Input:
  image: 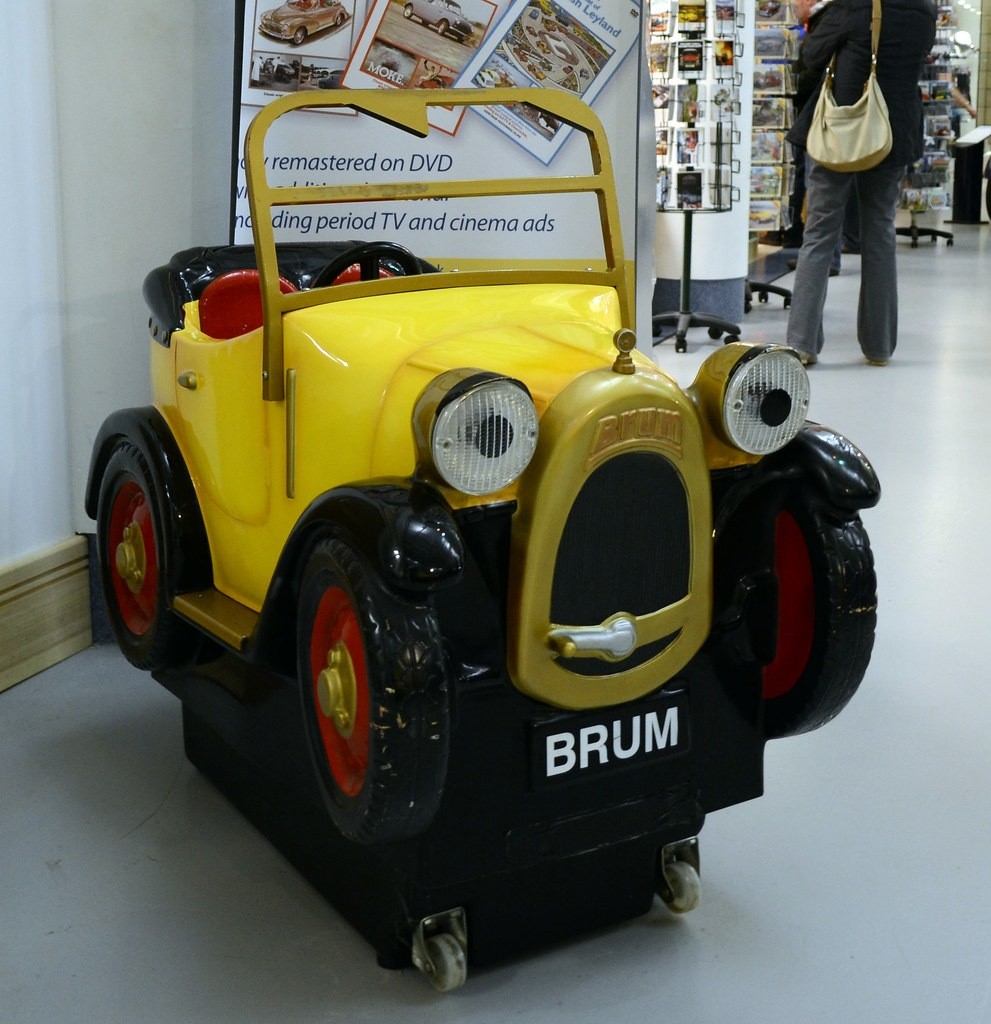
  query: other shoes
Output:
[841,244,858,253]
[870,360,887,366]
[786,258,839,276]
[796,349,818,365]
[759,234,802,247]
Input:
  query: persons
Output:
[759,0,937,365]
[946,82,978,118]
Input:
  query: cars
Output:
[83,86,880,852]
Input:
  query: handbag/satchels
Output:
[806,69,892,173]
